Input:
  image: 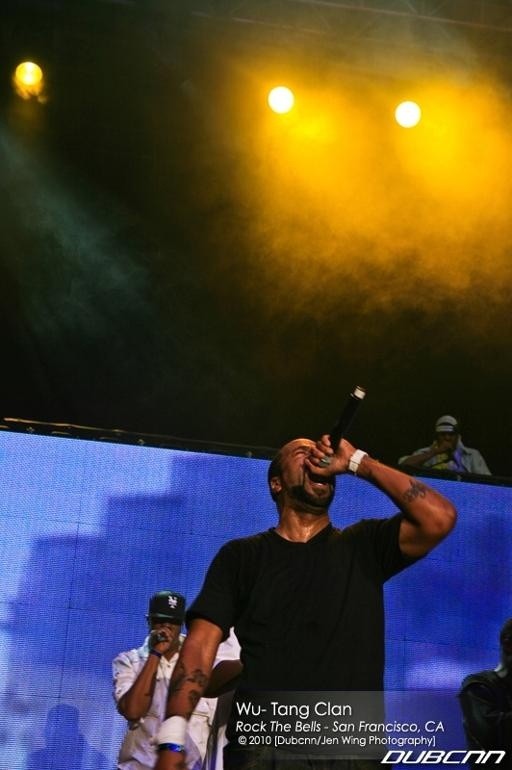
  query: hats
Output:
[143,590,187,625]
[434,413,459,434]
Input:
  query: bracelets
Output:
[149,648,162,659]
[154,715,190,745]
[154,743,189,757]
[347,448,369,477]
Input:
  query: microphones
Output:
[153,632,166,643]
[319,386,366,466]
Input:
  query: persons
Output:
[111,587,242,770]
[153,431,458,770]
[396,413,495,483]
[458,621,511,769]
